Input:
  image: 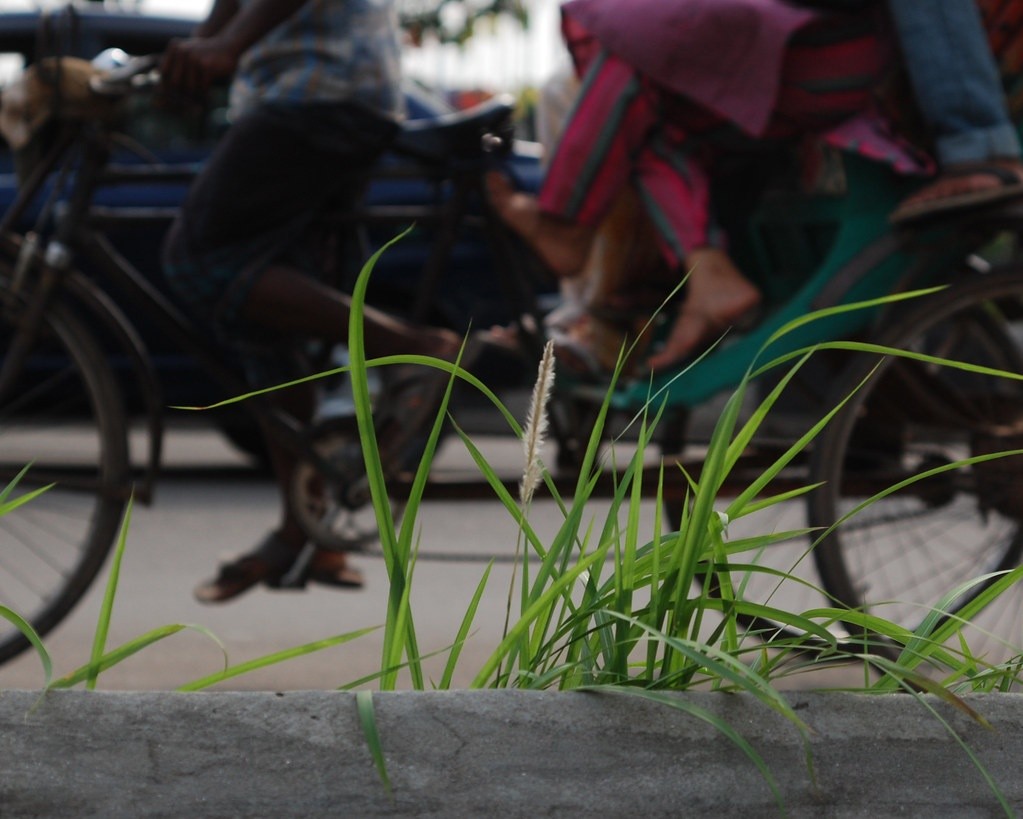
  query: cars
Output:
[1,8,554,415]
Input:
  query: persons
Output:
[484,0,1023,371]
[151,0,470,606]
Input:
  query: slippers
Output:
[340,334,480,515]
[194,521,369,606]
[884,161,1023,225]
[631,303,766,381]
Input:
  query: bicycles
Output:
[0,76,1023,671]
